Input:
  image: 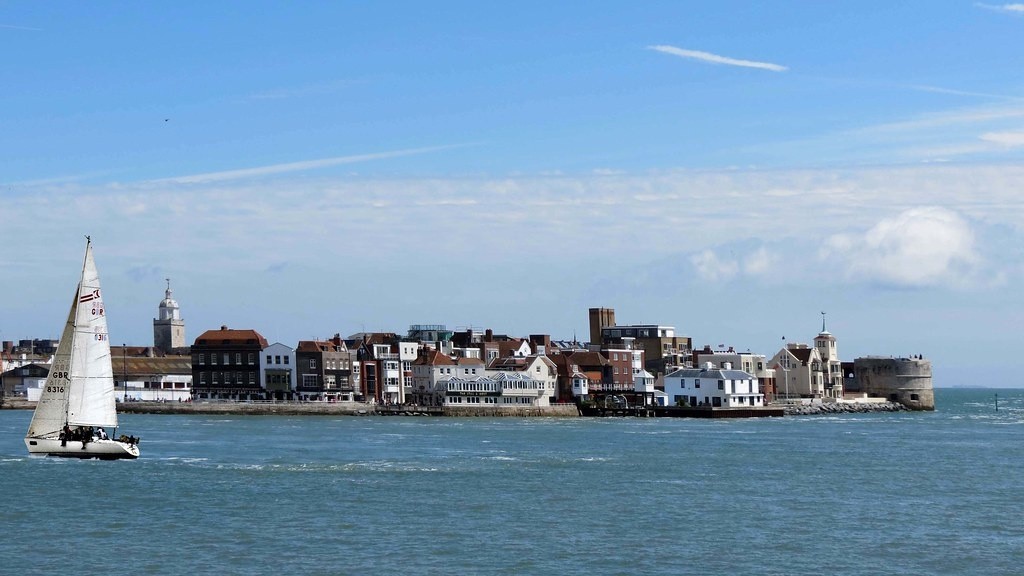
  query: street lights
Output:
[123,343,130,404]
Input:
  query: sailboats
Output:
[21,232,141,458]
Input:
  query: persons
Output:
[60,426,136,444]
[129,395,191,403]
